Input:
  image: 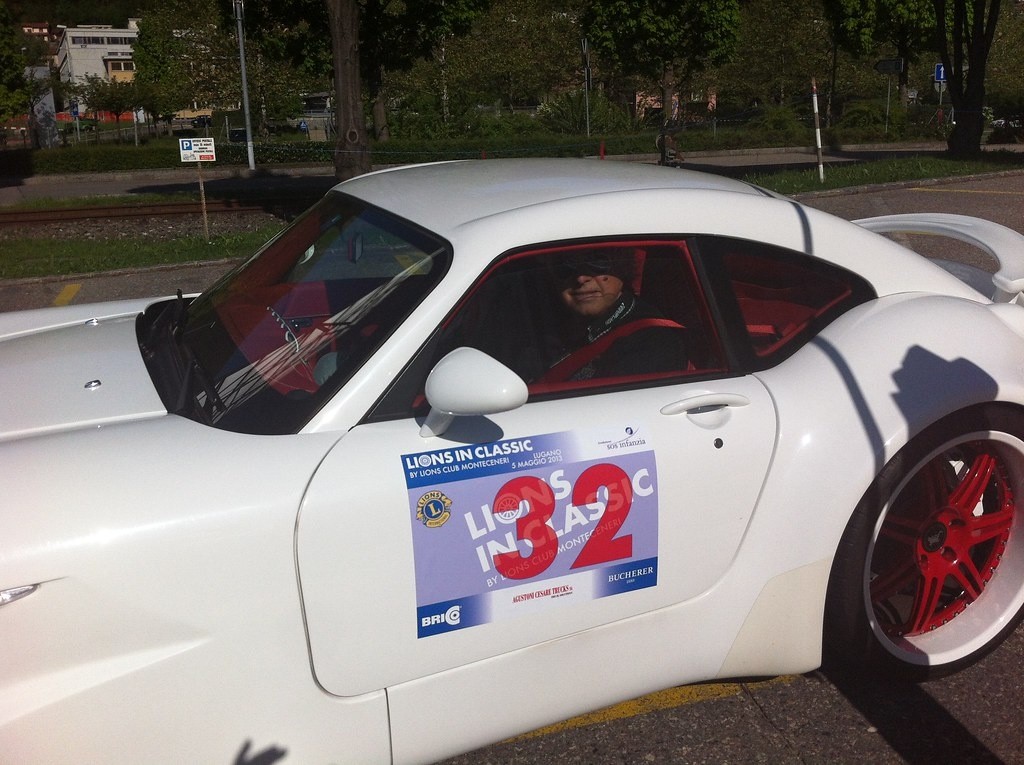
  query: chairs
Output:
[640,249,726,369]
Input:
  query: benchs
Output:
[723,253,838,354]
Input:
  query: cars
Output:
[990,115,1023,129]
[191,115,214,128]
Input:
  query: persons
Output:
[506,244,691,380]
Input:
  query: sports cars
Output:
[0,156,1024,765]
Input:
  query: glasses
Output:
[547,255,613,279]
